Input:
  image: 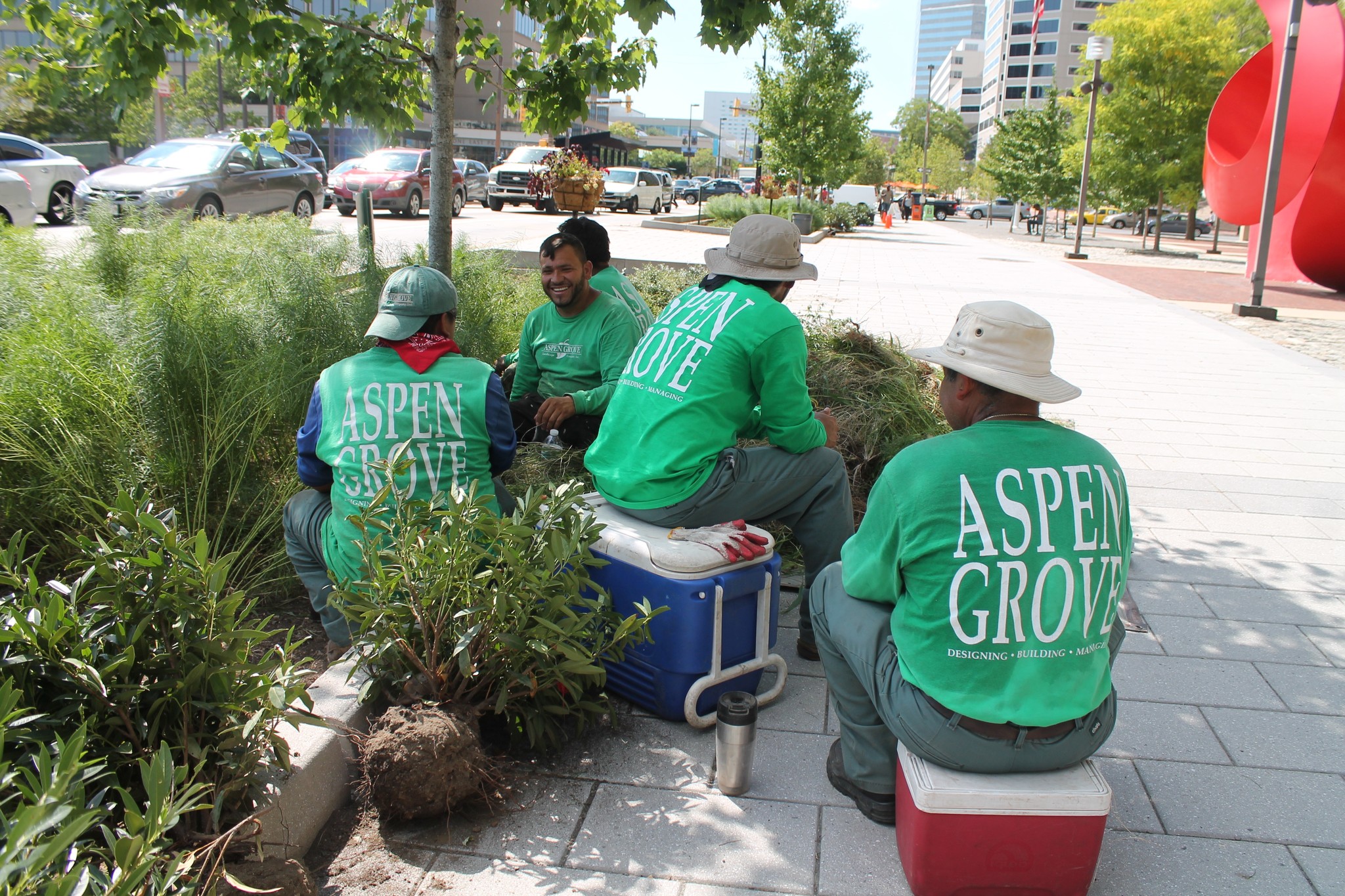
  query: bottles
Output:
[541,429,563,458]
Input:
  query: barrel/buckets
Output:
[792,213,813,235]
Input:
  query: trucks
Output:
[737,167,759,191]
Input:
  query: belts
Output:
[923,690,1084,741]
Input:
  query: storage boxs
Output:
[895,741,1112,895]
[534,493,787,728]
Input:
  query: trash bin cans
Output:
[792,212,813,235]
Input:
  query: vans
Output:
[831,185,878,225]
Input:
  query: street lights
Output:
[717,117,727,178]
[1065,35,1118,259]
[921,63,935,192]
[686,104,700,177]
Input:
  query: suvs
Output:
[680,178,748,204]
[486,146,564,214]
[205,127,328,188]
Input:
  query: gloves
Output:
[671,518,767,562]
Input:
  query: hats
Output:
[362,264,458,341]
[704,215,819,281]
[905,300,1082,404]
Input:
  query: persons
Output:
[1209,211,1215,229]
[586,213,855,658]
[807,300,1132,828]
[901,190,915,223]
[495,219,654,450]
[878,184,893,219]
[1024,204,1044,234]
[673,186,678,209]
[821,187,826,199]
[282,266,516,663]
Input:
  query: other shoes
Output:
[796,621,822,662]
[825,740,898,826]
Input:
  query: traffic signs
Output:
[917,167,933,173]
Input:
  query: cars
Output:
[0,133,91,233]
[328,146,491,219]
[963,197,1032,219]
[672,176,713,196]
[594,166,674,215]
[1066,206,1212,238]
[74,138,326,224]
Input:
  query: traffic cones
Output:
[881,212,892,229]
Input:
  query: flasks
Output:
[716,691,758,796]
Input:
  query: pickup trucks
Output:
[893,192,958,221]
